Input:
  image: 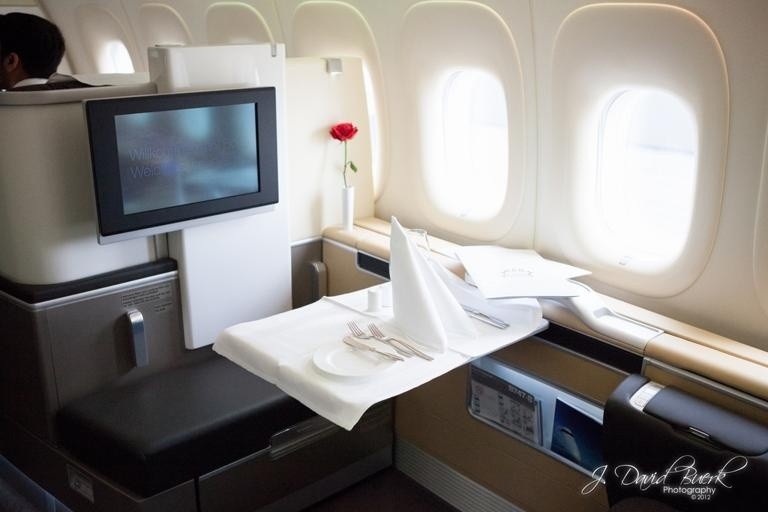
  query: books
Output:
[470,367,546,445]
[550,397,606,481]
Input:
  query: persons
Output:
[1,11,65,89]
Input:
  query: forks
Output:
[367,322,433,361]
[348,320,412,358]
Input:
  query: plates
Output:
[312,342,403,378]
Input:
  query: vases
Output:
[341,187,355,230]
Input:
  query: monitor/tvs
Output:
[84,86,279,245]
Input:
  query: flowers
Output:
[329,122,360,188]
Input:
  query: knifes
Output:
[343,335,404,361]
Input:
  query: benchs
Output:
[51,352,396,510]
